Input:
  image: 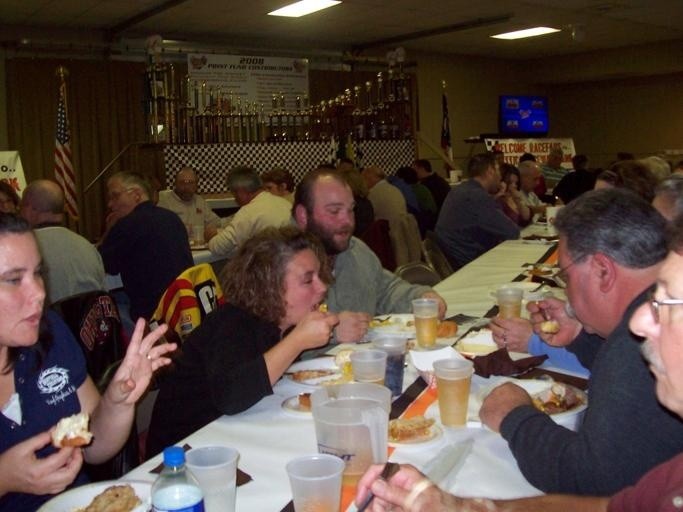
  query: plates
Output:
[279,393,313,417]
[38,481,156,511]
[458,329,498,357]
[386,420,442,448]
[284,357,346,387]
[502,377,587,420]
[366,313,415,339]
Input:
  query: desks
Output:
[44,178,588,511]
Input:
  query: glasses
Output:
[644,289,681,326]
[550,254,591,290]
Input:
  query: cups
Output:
[411,296,440,349]
[370,327,404,397]
[496,286,524,319]
[350,349,385,384]
[287,454,345,511]
[546,205,560,236]
[182,445,238,512]
[431,357,472,427]
[309,381,392,512]
[450,171,462,183]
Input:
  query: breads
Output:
[51,413,94,449]
[86,485,138,512]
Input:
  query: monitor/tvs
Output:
[498,95,549,135]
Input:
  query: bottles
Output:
[350,106,413,141]
[151,445,204,512]
[191,207,204,246]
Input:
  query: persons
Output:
[355,146,683,512]
[147,225,340,461]
[0,168,218,511]
[279,166,446,364]
[205,165,296,257]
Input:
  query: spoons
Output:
[535,300,561,334]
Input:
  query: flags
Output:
[54,84,79,222]
[441,93,454,170]
